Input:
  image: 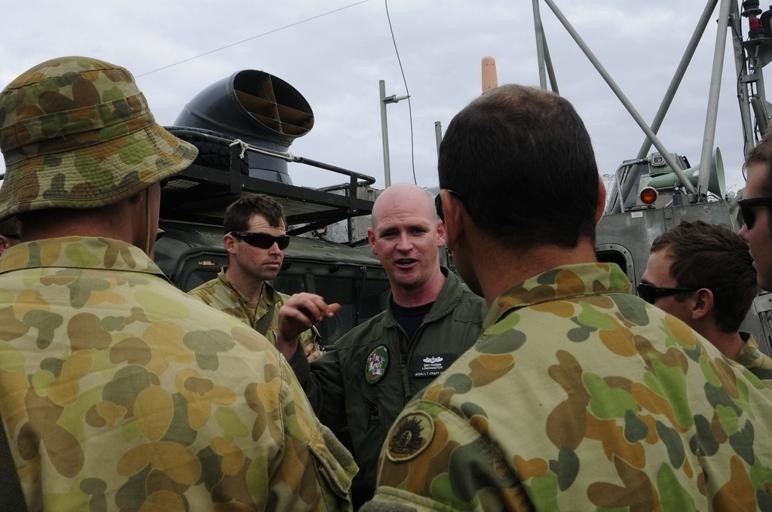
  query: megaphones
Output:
[647,146,726,200]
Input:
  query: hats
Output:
[1,55,200,238]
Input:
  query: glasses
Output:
[232,231,291,249]
[636,283,697,304]
[736,197,770,230]
[433,189,463,223]
